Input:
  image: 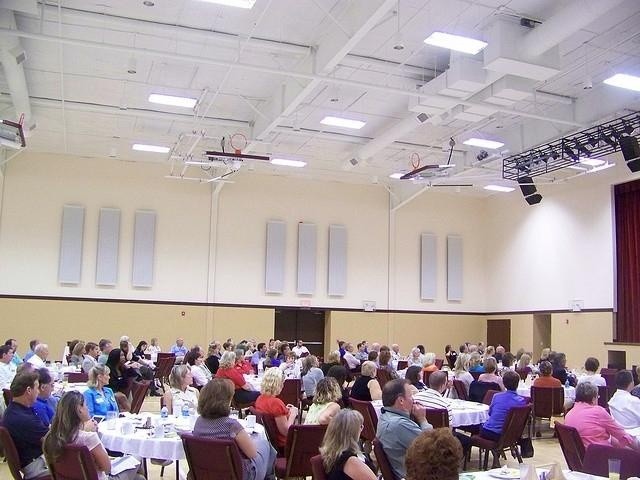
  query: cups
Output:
[608,458,621,479]
[230,410,238,422]
[149,426,164,439]
[61,377,68,389]
[106,410,117,430]
[120,421,134,434]
[245,414,257,428]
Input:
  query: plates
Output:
[489,467,522,479]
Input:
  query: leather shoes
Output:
[150,392,162,396]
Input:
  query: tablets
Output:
[91,415,105,424]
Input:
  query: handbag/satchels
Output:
[521,437,534,459]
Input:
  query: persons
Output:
[1,334,640,479]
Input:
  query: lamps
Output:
[513,119,640,205]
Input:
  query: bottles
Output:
[182,403,190,417]
[161,404,169,422]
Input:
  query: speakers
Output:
[517,176,537,196]
[524,194,543,205]
[619,136,640,161]
[627,159,640,172]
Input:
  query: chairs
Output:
[0,346,640,480]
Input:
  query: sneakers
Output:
[152,458,172,467]
